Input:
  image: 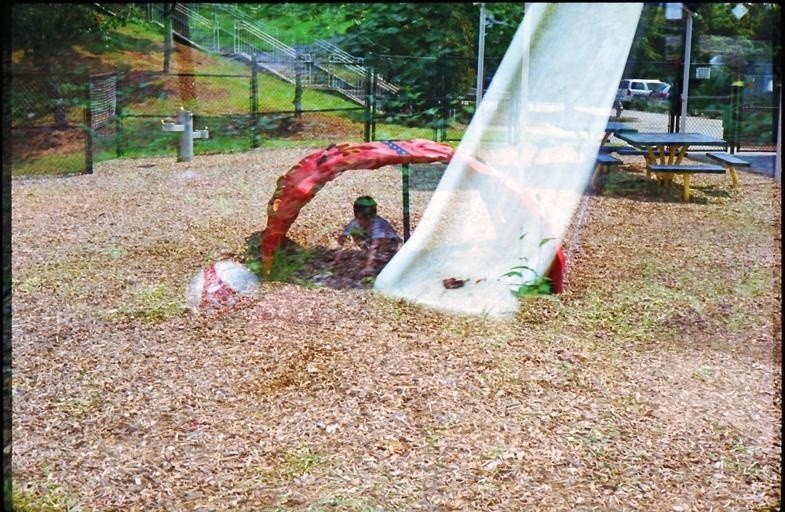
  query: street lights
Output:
[659,1,696,135]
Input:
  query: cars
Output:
[480,72,496,91]
[656,85,670,99]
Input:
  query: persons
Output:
[331,196,398,276]
[668,79,683,133]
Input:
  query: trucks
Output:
[678,58,776,118]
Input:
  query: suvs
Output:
[616,78,670,109]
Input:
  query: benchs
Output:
[594,143,751,203]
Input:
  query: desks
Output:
[604,122,638,144]
[615,131,728,193]
